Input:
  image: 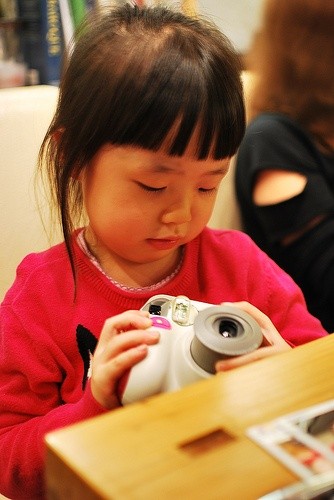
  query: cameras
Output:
[115,293,267,406]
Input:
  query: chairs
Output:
[0,85,90,307]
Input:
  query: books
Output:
[14,0,206,87]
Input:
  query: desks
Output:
[44,333,334,500]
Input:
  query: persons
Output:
[235,1,334,334]
[0,0,331,500]
